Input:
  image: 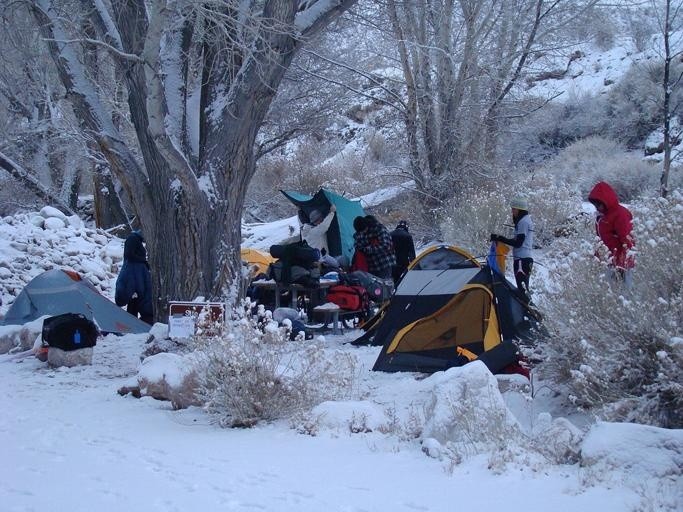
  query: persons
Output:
[587,180,635,288]
[352,215,397,279]
[298,204,336,258]
[490,197,535,294]
[246,263,273,304]
[115,228,153,323]
[389,219,416,277]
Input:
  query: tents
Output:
[372,263,542,375]
[350,243,482,346]
[1,268,152,336]
[279,187,366,267]
[241,247,279,277]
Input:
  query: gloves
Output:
[490,233,498,242]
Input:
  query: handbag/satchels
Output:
[40,313,98,352]
[265,240,391,311]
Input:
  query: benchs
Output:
[312,304,377,336]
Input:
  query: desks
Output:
[251,279,338,310]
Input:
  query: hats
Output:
[309,210,322,224]
[510,193,531,211]
[396,220,409,232]
